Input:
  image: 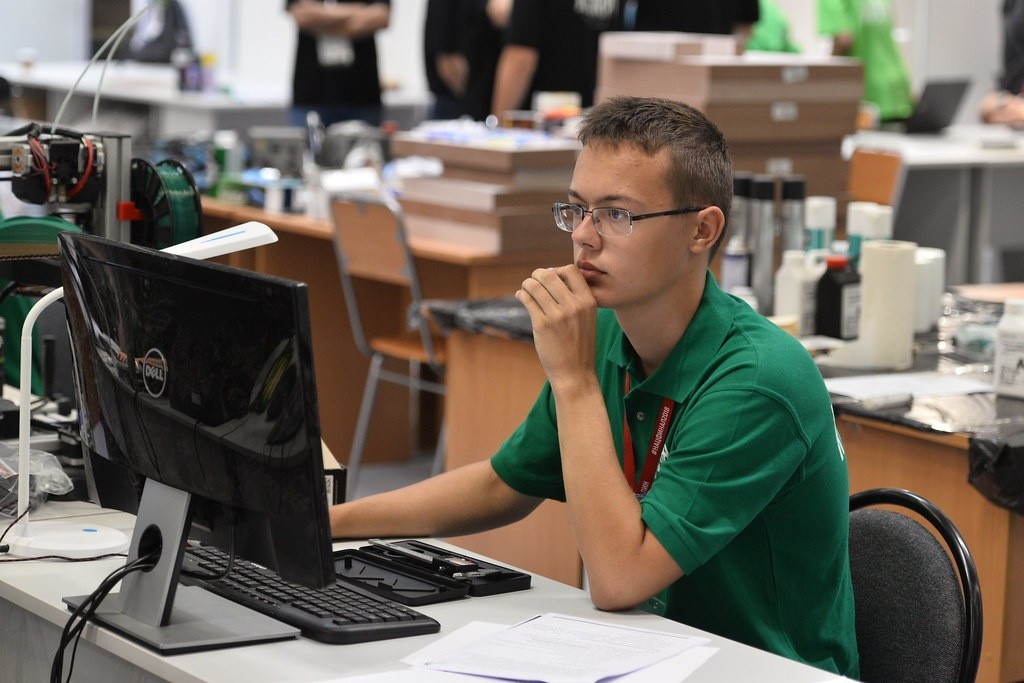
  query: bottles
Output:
[995,299,1024,400]
[722,235,757,318]
[863,206,893,240]
[775,241,858,339]
[846,201,879,269]
[804,195,835,252]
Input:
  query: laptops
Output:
[882,81,970,134]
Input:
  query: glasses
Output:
[550,201,707,237]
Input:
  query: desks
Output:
[201,198,627,468]
[429,277,1024,683]
[0,435,855,683]
[0,60,238,160]
[938,118,1024,280]
[852,122,973,286]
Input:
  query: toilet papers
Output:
[856,238,919,369]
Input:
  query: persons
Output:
[818,0,915,129]
[329,97,862,680]
[284,0,391,123]
[424,0,802,124]
[984,0,1024,128]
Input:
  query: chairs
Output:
[316,185,452,489]
[845,485,983,683]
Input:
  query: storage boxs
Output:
[581,39,873,147]
[394,127,580,179]
[728,146,851,220]
[391,188,568,217]
[402,200,570,255]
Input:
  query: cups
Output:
[917,244,944,334]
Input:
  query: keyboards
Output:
[173,544,442,644]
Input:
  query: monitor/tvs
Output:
[59,232,338,657]
